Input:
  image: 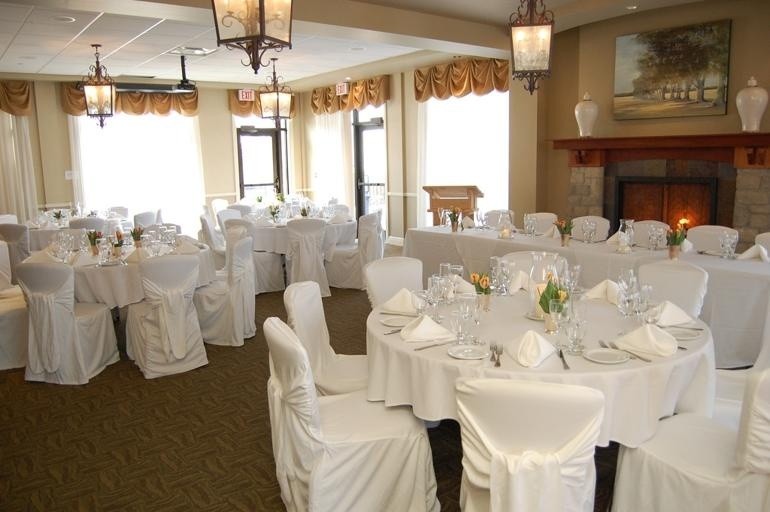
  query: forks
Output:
[495,344,503,367]
[610,342,651,362]
[489,341,497,362]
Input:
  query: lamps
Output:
[79,44,115,128]
[509,0,555,95]
[211,0,293,74]
[258,58,293,122]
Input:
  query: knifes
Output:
[556,346,572,371]
[412,339,458,353]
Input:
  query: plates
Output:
[583,348,631,364]
[380,316,411,327]
[667,327,702,340]
[448,345,490,360]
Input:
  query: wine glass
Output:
[411,252,662,348]
[256,198,334,223]
[55,226,177,264]
[438,207,738,260]
